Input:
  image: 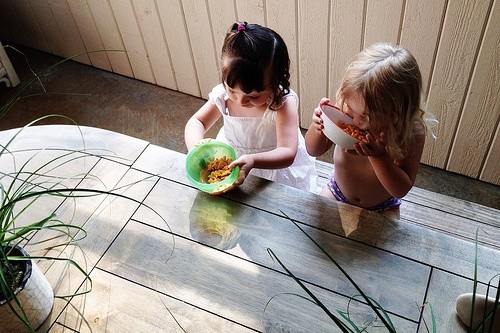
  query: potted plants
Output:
[0,114,176,333]
[450,225,500,333]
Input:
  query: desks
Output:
[0,123,500,333]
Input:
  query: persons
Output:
[305,43,428,219]
[183,22,320,189]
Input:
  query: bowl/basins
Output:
[185,138,238,193]
[319,101,374,151]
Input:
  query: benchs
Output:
[309,158,498,250]
